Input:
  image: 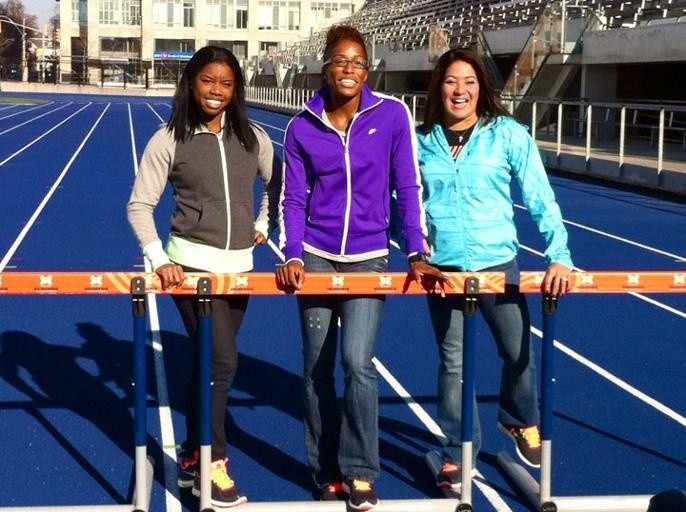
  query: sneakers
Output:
[497,420,542,468]
[437,462,477,490]
[177,450,249,507]
[319,478,379,510]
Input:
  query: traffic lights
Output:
[55,28,61,44]
[44,24,49,37]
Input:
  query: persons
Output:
[274,27,456,509]
[392,47,577,493]
[126,47,283,509]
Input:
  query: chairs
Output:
[258,2,686,70]
[565,99,686,153]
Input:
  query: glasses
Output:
[320,55,370,70]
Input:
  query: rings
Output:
[563,277,568,281]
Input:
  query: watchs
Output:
[406,252,429,265]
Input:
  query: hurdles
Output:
[519,270,686,512]
[0,270,507,512]
